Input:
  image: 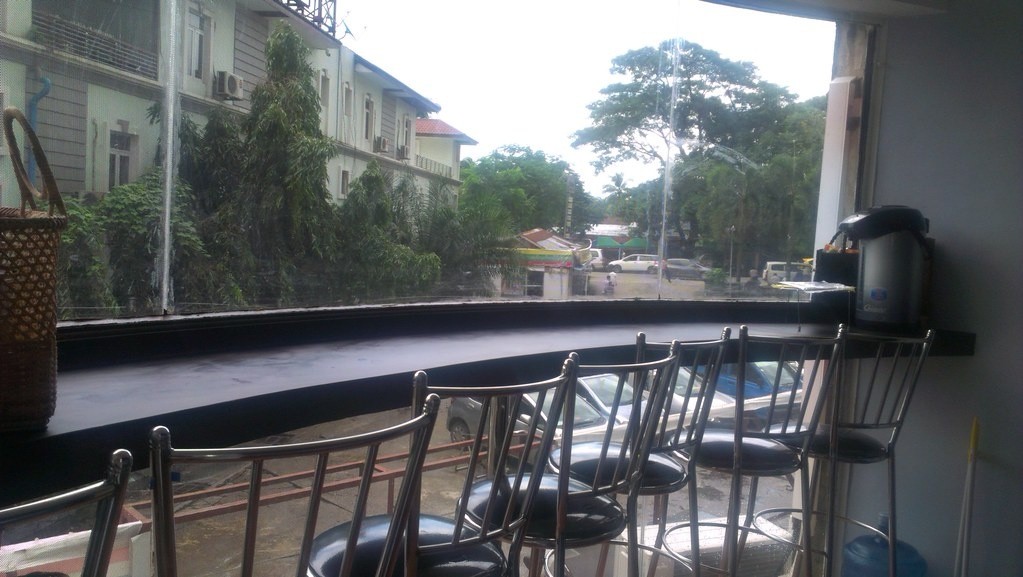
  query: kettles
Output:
[835,203,938,339]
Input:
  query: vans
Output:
[764,261,813,286]
[587,248,608,272]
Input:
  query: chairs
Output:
[16,328,940,576]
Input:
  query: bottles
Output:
[843,511,928,577]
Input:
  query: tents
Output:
[592,236,652,266]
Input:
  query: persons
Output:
[610,269,618,288]
[605,275,613,289]
[662,255,672,283]
[605,281,614,297]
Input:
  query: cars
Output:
[608,253,664,274]
[799,258,814,271]
[665,258,715,281]
